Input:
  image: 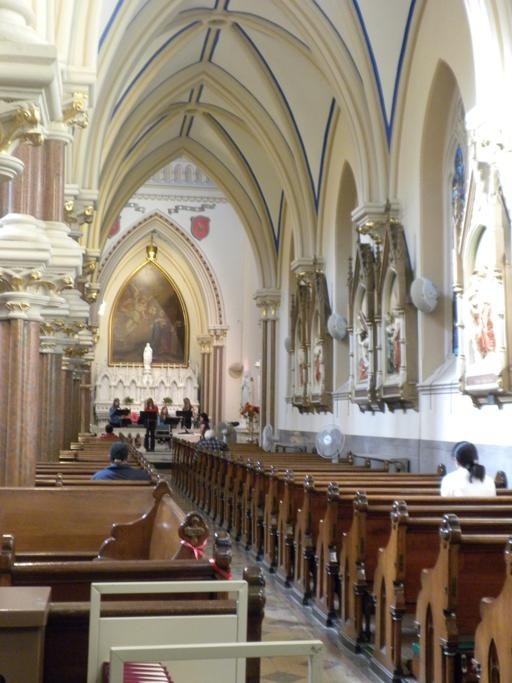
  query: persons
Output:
[439,441,499,497]
[117,283,185,357]
[90,397,230,480]
[142,341,154,369]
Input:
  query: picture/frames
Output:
[108,259,191,367]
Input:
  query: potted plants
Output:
[161,396,174,420]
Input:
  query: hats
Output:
[110,442,129,457]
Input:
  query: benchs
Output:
[1,565,267,683]
[0,436,236,600]
[169,436,511,683]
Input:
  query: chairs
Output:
[108,639,323,683]
[85,578,250,683]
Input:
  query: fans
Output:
[259,423,274,452]
[215,420,240,445]
[313,423,346,464]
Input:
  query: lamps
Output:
[145,229,158,261]
